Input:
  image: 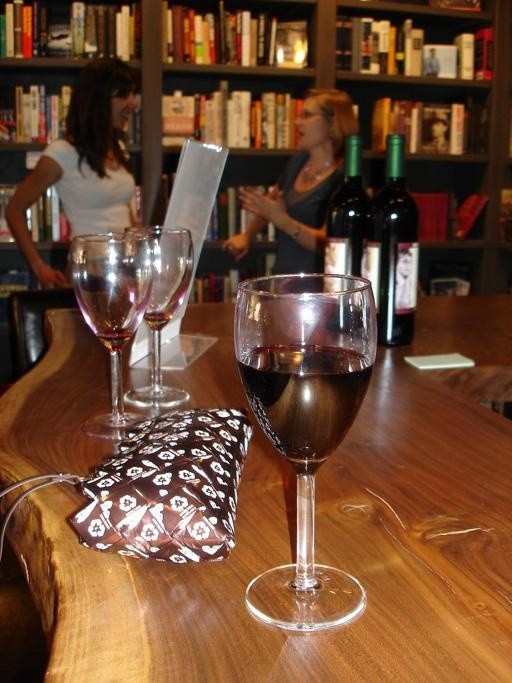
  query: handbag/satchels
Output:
[66,402,252,564]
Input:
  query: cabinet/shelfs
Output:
[1,0,512,319]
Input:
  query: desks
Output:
[2,295,512,683]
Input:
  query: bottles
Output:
[321,132,372,333]
[356,131,422,347]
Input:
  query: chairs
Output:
[13,289,83,370]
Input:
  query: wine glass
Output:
[230,272,381,637]
[67,231,156,442]
[121,224,197,408]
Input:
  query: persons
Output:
[3,56,148,290]
[223,88,361,279]
